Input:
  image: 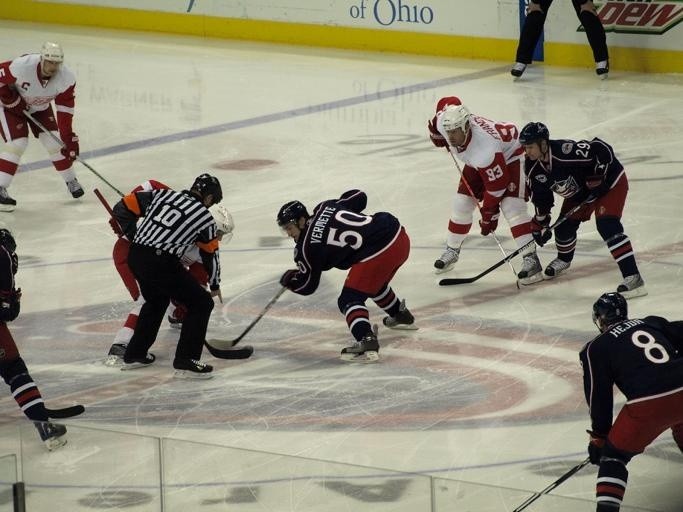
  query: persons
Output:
[579,293,683,512]
[112,173,222,375]
[109,180,235,357]
[0,39,84,206]
[518,121,645,292]
[511,0,610,76]
[276,189,415,355]
[0,229,67,441]
[429,96,543,278]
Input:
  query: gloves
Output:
[279,270,299,287]
[1,90,32,117]
[479,205,500,235]
[61,133,79,161]
[529,215,552,247]
[586,426,612,464]
[430,123,450,147]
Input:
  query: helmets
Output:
[277,201,306,226]
[592,292,627,324]
[40,40,63,63]
[519,122,549,144]
[441,105,470,132]
[191,173,235,237]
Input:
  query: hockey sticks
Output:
[439,205,579,286]
[207,286,290,349]
[46,404,85,420]
[513,456,590,512]
[93,188,253,360]
[22,109,124,197]
[449,150,521,288]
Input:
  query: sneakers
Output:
[66,178,84,198]
[511,62,527,77]
[595,59,608,74]
[0,187,16,205]
[383,299,413,326]
[617,273,644,291]
[434,246,460,269]
[341,324,379,353]
[108,343,213,373]
[518,252,571,278]
[34,423,67,441]
